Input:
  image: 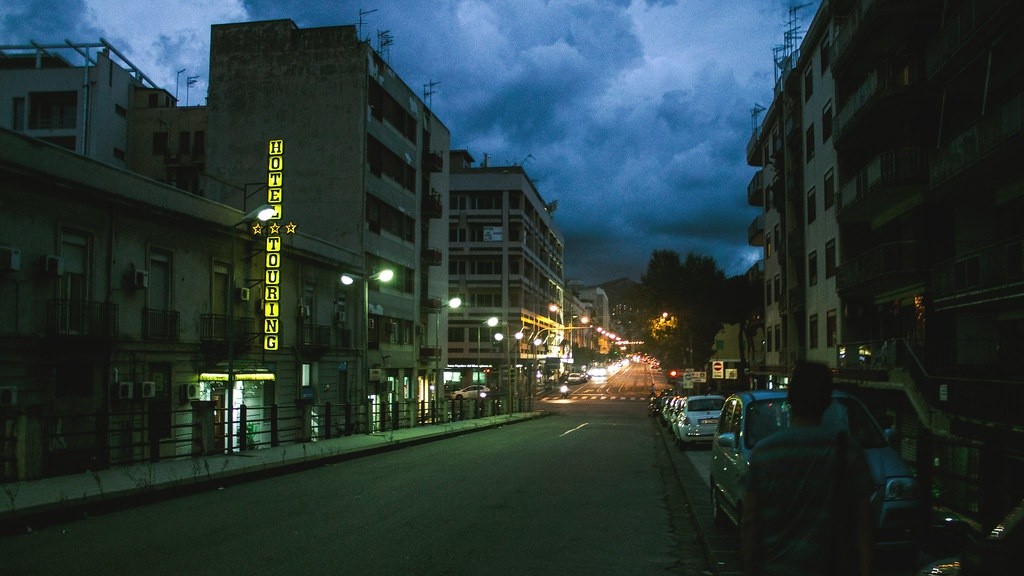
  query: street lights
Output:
[435,297,463,425]
[514,333,524,413]
[662,311,693,367]
[670,371,678,395]
[494,333,513,414]
[533,338,543,400]
[336,268,396,437]
[476,317,499,419]
[227,203,276,458]
[549,304,558,346]
[569,315,621,374]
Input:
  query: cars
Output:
[594,366,607,378]
[567,372,581,385]
[916,500,1024,576]
[642,355,660,368]
[661,394,729,449]
[710,390,921,561]
[450,385,491,400]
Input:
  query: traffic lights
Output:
[483,367,490,373]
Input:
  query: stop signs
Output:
[713,361,724,373]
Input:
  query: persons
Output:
[739,358,876,576]
